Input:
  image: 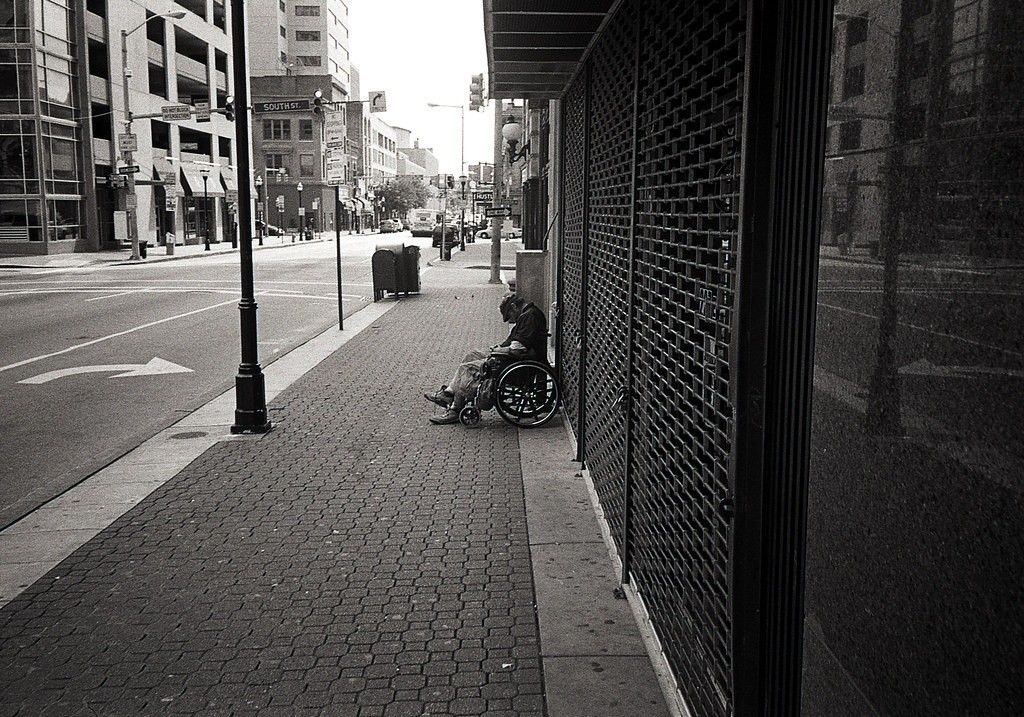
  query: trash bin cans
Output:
[440,245,451,261]
[139,240,148,258]
[305,231,311,240]
[405,245,421,292]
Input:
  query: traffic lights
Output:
[312,89,324,115]
[468,73,484,111]
[226,94,235,121]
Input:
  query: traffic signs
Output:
[119,165,140,174]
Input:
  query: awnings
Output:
[153,160,258,198]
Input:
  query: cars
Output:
[255,220,286,236]
[378,208,522,248]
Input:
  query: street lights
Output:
[469,180,478,243]
[120,9,187,262]
[427,102,464,176]
[255,174,264,245]
[459,175,467,252]
[296,181,304,240]
[200,164,211,251]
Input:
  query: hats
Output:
[499,293,518,322]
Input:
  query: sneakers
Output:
[424,385,455,408]
[429,403,461,424]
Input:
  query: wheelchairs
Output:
[434,328,563,430]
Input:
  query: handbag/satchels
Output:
[479,380,495,411]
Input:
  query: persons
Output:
[424,292,547,425]
